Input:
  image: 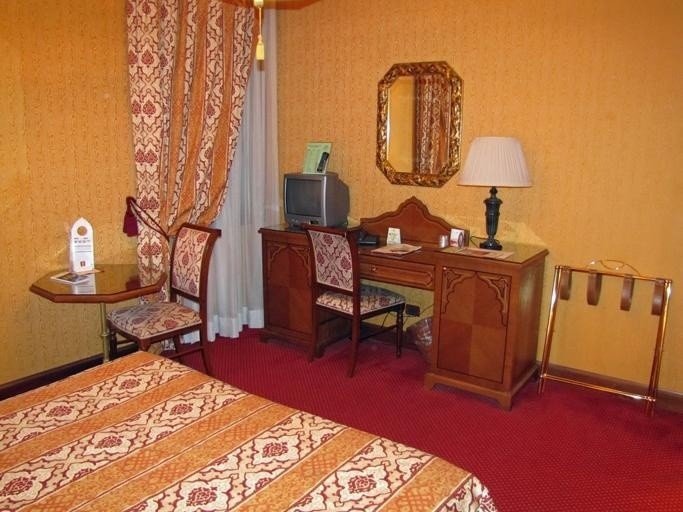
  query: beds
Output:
[0,348,499,511]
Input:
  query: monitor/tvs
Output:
[282,173,351,233]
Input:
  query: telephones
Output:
[356,231,378,245]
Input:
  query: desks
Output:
[256,195,550,411]
[28,263,166,365]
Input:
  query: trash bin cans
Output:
[407,316,432,364]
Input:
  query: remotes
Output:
[316,150,330,173]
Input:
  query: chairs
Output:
[103,221,222,376]
[299,223,407,376]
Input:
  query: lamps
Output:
[459,137,530,250]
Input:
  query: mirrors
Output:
[374,60,465,188]
